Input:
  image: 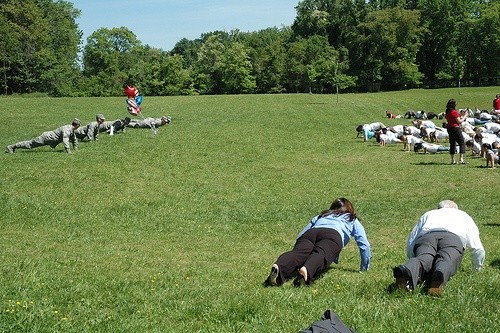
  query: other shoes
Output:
[7,145,13,154]
[426,269,444,296]
[393,265,409,294]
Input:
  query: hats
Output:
[73,118,81,126]
[161,116,167,122]
[96,114,105,119]
[167,116,172,121]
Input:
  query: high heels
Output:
[264,266,278,286]
[292,268,305,288]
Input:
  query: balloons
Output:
[126,85,142,116]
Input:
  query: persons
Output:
[393,199,485,298]
[356,110,460,154]
[7,118,81,154]
[442,94,500,169]
[266,198,370,287]
[445,99,467,164]
[42,114,171,143]
